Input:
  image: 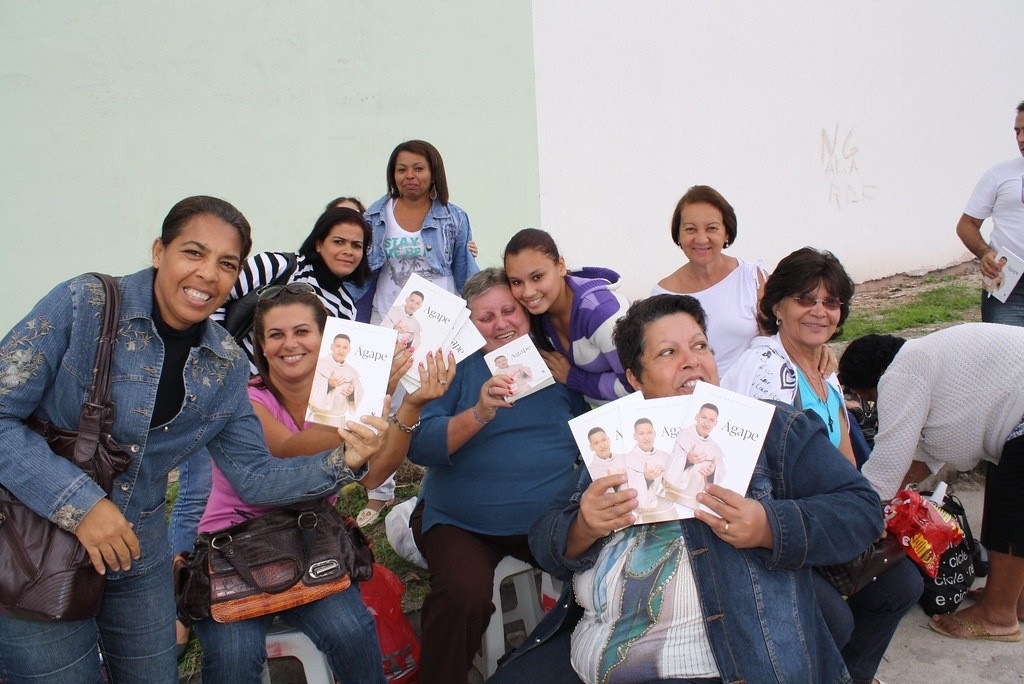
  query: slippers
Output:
[929,611,1023,642]
[967,587,1024,622]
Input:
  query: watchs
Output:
[393,412,421,433]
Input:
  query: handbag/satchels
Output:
[358,563,421,684]
[0,271,132,622]
[385,496,428,570]
[207,497,375,624]
[884,481,989,617]
[842,385,879,450]
[173,550,210,628]
[812,501,907,601]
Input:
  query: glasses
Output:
[258,282,317,303]
[790,294,845,311]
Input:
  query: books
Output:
[567,381,776,533]
[379,273,487,395]
[484,335,556,404]
[304,315,398,435]
[980,247,1024,304]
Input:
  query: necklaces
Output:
[784,347,834,432]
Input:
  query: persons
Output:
[504,229,632,408]
[494,355,532,399]
[982,256,1007,294]
[379,291,424,360]
[486,294,884,684]
[407,267,582,684]
[308,333,365,430]
[324,140,482,326]
[623,418,677,525]
[587,417,626,531]
[651,100,1024,684]
[657,403,724,509]
[0,196,388,684]
[168,206,371,667]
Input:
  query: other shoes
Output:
[356,498,395,528]
[176,642,189,663]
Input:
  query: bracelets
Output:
[473,405,490,425]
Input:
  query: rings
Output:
[488,387,493,397]
[437,379,449,385]
[724,522,729,533]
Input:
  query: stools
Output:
[471,555,561,682]
[259,615,336,684]
